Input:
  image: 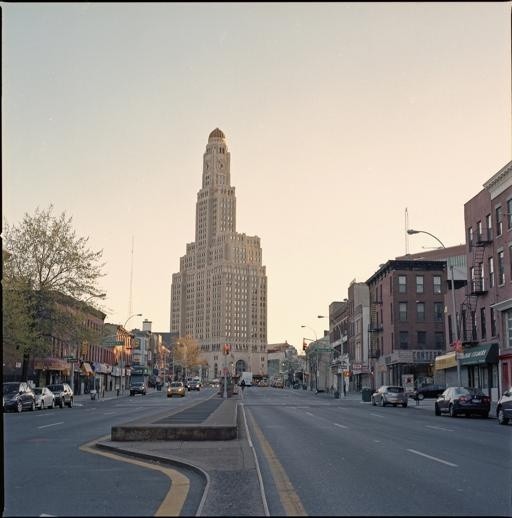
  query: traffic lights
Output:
[303,340,307,350]
[224,342,230,355]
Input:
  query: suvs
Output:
[369,385,408,408]
[2,381,36,412]
[186,375,220,391]
[45,382,74,409]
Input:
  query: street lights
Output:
[120,314,142,395]
[162,342,180,388]
[318,314,346,401]
[75,293,106,366]
[407,229,463,386]
[301,325,317,341]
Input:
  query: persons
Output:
[240,379,245,391]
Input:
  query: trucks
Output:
[236,370,253,385]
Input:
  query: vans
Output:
[270,374,284,388]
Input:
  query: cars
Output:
[435,386,491,419]
[32,386,55,409]
[257,380,269,386]
[497,386,512,426]
[167,381,185,397]
[409,383,445,400]
[130,382,146,396]
